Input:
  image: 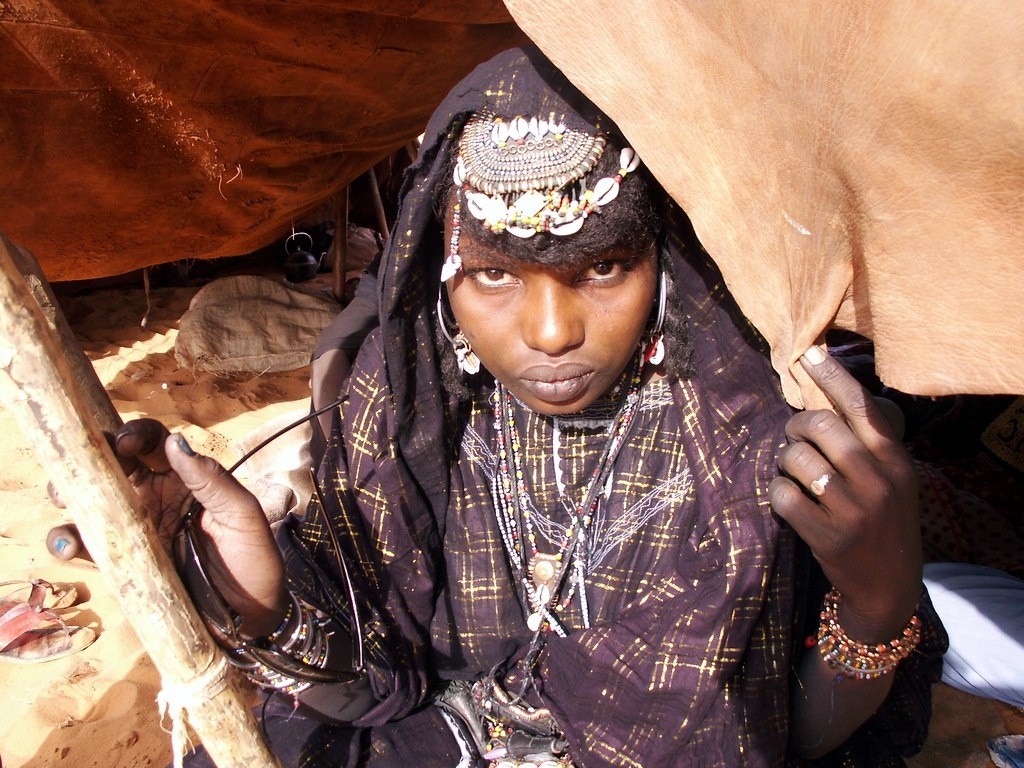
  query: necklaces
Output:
[480,340,646,639]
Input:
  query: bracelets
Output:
[208,578,333,698]
[816,587,923,686]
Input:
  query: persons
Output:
[28,47,953,768]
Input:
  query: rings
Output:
[811,470,838,498]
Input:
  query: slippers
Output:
[0,578,96,663]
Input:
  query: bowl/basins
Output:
[986,734,1024,768]
